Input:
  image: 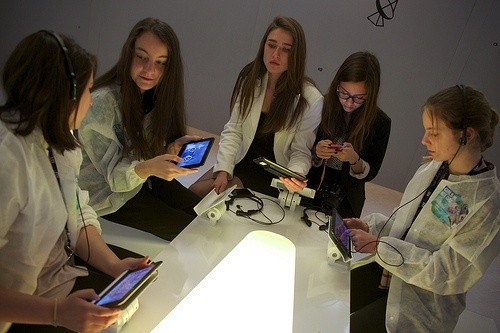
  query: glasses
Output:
[336,84,368,104]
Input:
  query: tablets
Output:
[253,158,306,181]
[174,137,215,168]
[332,207,353,258]
[90,261,162,310]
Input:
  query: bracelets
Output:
[53,298,57,327]
[349,156,361,166]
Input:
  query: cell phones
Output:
[232,188,255,197]
[328,144,342,154]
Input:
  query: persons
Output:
[189,16,324,199]
[0,30,149,333]
[78,18,205,229]
[342,84,500,333]
[301,52,392,218]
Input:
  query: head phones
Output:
[457,84,466,145]
[301,206,329,230]
[40,30,77,101]
[225,196,263,216]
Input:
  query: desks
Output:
[102,190,350,333]
